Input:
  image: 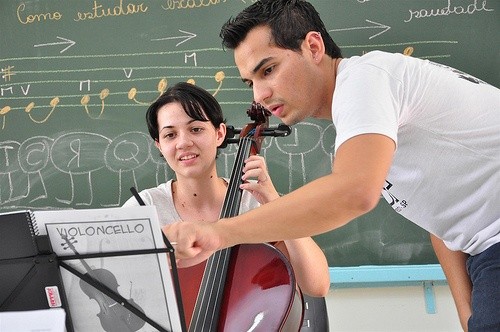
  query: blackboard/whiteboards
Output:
[0,1,500,286]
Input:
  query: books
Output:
[0,208,41,261]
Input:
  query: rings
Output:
[170,242,177,245]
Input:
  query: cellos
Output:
[170,101,305,332]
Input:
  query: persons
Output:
[158,0,500,332]
[118,82,331,298]
